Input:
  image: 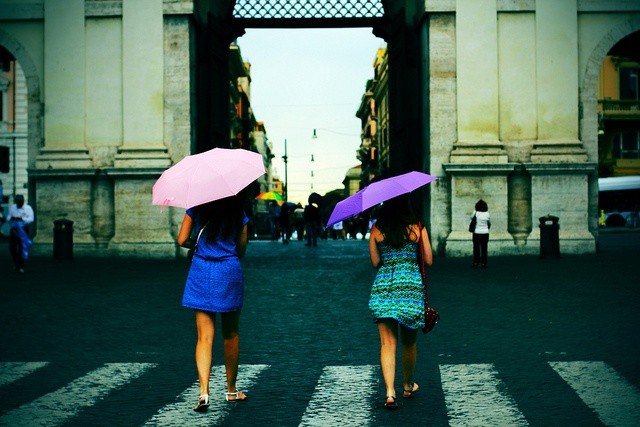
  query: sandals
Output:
[224,389,247,402]
[401,382,419,398]
[383,396,398,409]
[193,393,210,410]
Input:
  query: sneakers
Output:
[305,243,311,246]
[314,243,317,247]
[471,263,478,268]
[480,264,487,269]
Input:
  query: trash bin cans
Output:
[53,218,75,259]
[538,215,560,256]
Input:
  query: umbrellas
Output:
[255,193,284,200]
[323,167,438,230]
[152,143,268,212]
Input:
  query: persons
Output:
[469,198,493,267]
[254,201,332,246]
[176,193,250,413]
[365,193,433,410]
[6,192,35,276]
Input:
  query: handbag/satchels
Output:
[469,212,477,232]
[422,307,440,334]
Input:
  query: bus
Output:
[597,175,640,229]
